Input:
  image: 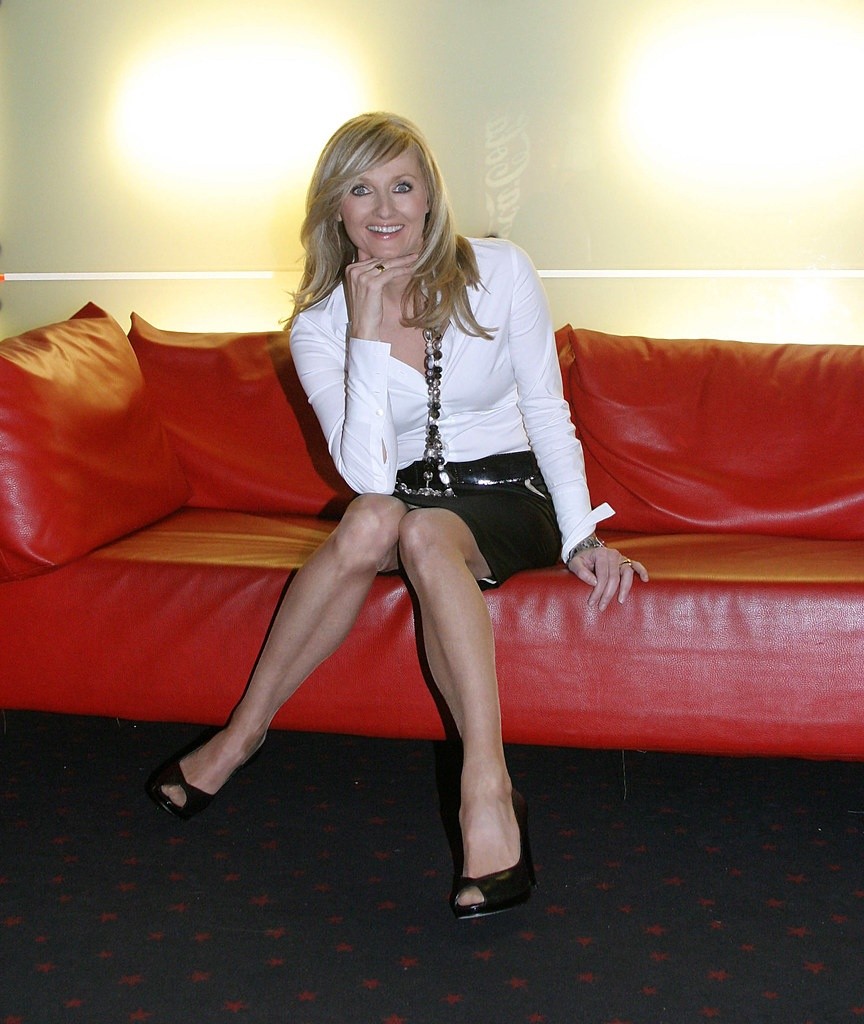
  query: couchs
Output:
[0,301,864,763]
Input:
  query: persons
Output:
[144,111,649,920]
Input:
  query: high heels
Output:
[145,731,266,823]
[451,787,538,920]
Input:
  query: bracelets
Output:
[567,536,605,562]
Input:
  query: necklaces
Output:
[395,299,458,498]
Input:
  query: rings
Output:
[375,264,385,273]
[619,559,632,571]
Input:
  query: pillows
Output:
[1,300,863,575]
